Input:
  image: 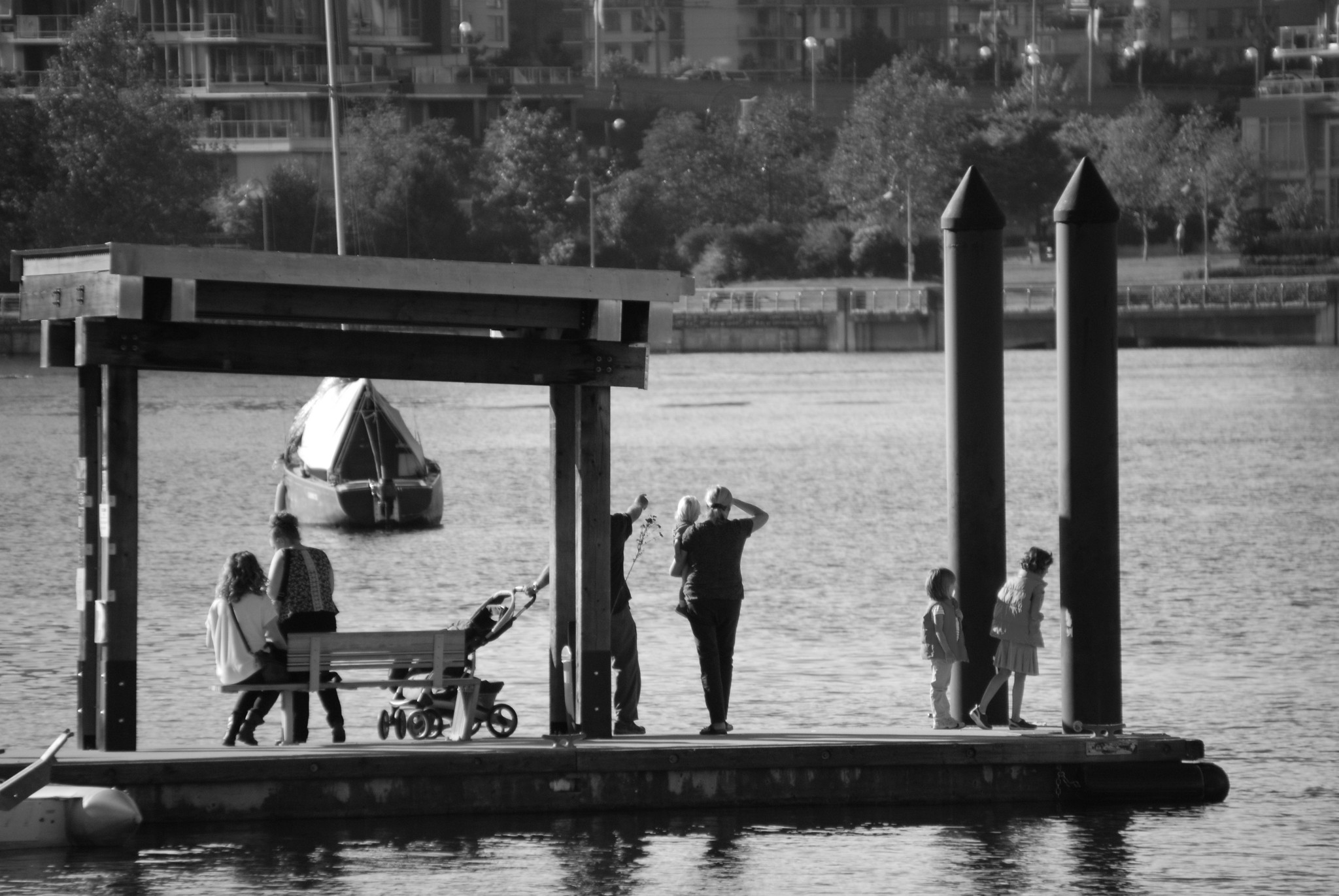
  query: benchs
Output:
[709,291,742,310]
[213,630,481,745]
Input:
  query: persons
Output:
[523,493,648,735]
[920,568,970,728]
[205,551,287,748]
[669,485,769,735]
[969,547,1054,730]
[264,511,346,746]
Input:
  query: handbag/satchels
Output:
[256,641,278,667]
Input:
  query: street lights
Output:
[881,166,914,291]
[1245,45,1259,98]
[801,35,818,109]
[1026,42,1041,111]
[566,173,597,268]
[1180,162,1209,284]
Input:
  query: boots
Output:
[222,709,245,746]
[237,711,265,745]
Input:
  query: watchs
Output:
[533,582,539,592]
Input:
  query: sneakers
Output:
[970,704,993,730]
[955,719,965,728]
[933,716,959,729]
[614,720,645,734]
[1008,719,1037,730]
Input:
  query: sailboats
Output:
[269,2,447,533]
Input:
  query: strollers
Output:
[375,584,537,742]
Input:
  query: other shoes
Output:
[725,721,733,731]
[675,604,689,619]
[332,726,346,743]
[700,724,727,735]
